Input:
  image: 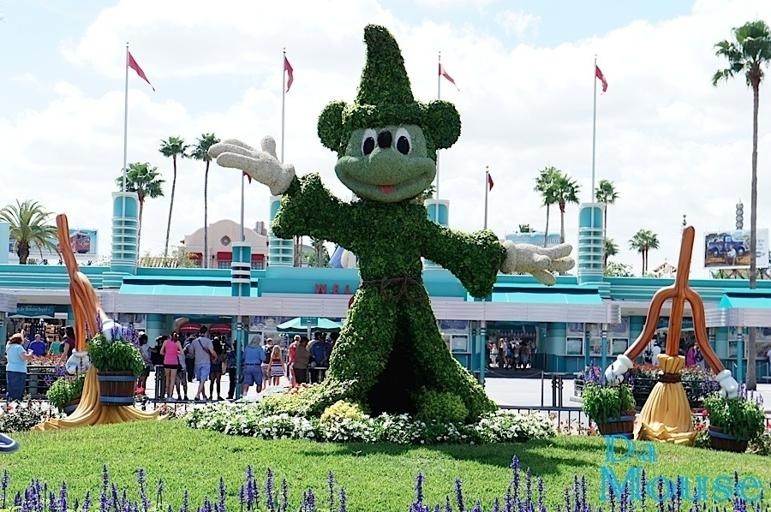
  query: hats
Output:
[318,333,327,336]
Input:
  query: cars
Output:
[709,235,749,257]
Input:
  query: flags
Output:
[243,170,253,184]
[596,66,609,95]
[438,64,455,84]
[488,172,494,192]
[128,53,155,91]
[283,57,295,94]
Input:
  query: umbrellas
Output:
[277,318,342,341]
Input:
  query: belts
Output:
[246,363,261,365]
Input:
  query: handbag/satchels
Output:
[211,355,216,364]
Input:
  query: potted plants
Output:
[702,392,765,453]
[87,333,146,405]
[582,381,637,440]
[46,371,86,416]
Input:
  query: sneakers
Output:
[194,394,225,402]
[164,395,188,402]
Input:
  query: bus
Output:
[57,232,92,254]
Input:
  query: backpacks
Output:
[263,345,274,364]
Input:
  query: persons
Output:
[646,332,704,368]
[137,324,339,402]
[766,350,771,367]
[5,324,74,400]
[487,336,534,370]
[724,244,738,266]
